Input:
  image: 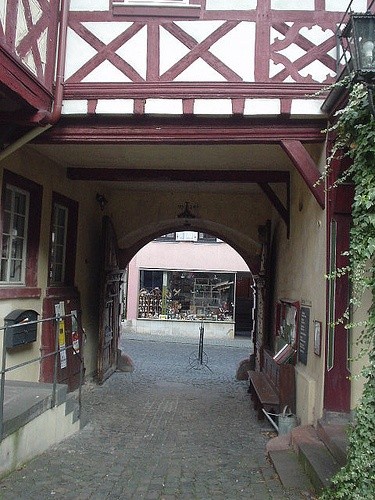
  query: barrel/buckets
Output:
[278,405,297,437]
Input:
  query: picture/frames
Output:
[312,320,322,357]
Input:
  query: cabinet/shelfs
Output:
[193,278,221,318]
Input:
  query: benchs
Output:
[246,348,296,421]
